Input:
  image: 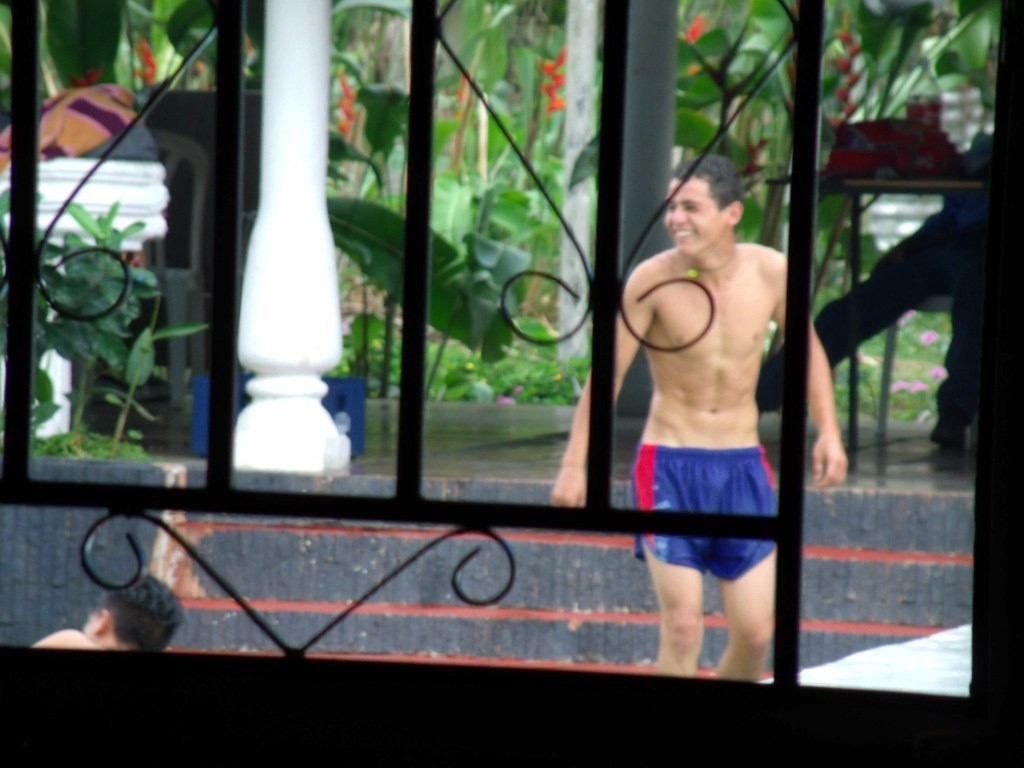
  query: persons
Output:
[753,125,993,448]
[26,572,183,651]
[551,153,847,680]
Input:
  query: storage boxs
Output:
[191,374,363,459]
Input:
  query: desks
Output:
[759,175,991,360]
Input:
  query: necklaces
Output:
[679,243,738,278]
